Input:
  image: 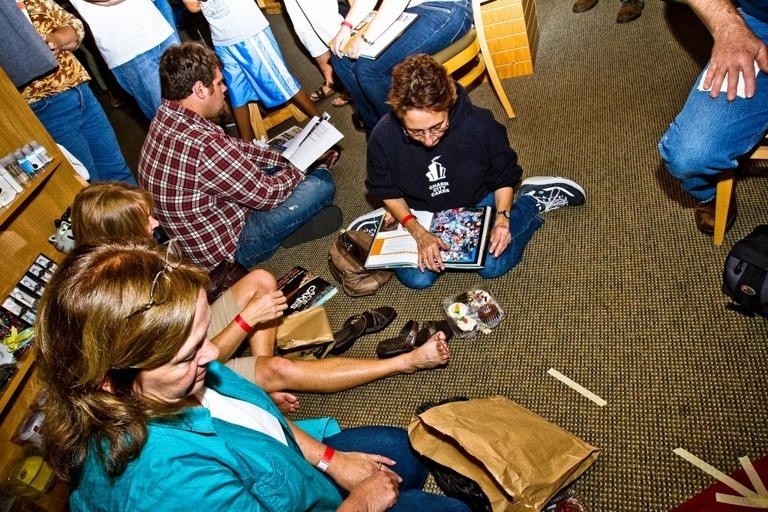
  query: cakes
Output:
[446,288,499,332]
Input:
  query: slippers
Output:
[282,204,345,250]
[315,144,342,170]
[324,306,399,356]
[376,314,453,360]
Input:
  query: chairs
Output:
[714,135,768,249]
[430,1,519,119]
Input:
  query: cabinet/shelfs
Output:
[0,67,91,512]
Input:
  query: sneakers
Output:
[346,206,384,234]
[517,174,587,212]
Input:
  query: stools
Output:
[248,101,308,143]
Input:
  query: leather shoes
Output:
[691,181,738,235]
[572,0,599,12]
[616,0,646,23]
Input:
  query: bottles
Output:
[0,139,54,185]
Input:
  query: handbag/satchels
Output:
[407,396,491,511]
[720,222,768,321]
[327,227,392,299]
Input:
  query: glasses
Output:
[123,237,183,320]
[400,117,449,139]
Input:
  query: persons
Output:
[656,0,768,236]
[0,1,645,511]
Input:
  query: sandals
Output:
[308,79,350,109]
